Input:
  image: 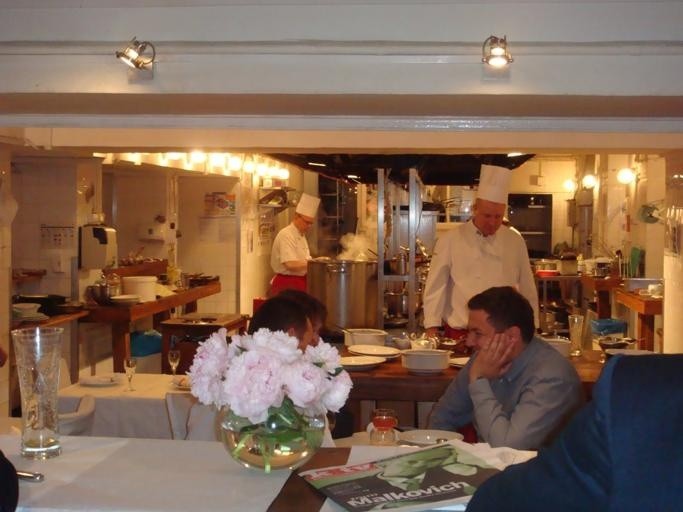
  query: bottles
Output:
[576,254,585,276]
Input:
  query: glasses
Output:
[299,216,313,226]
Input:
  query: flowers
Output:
[185,323,352,424]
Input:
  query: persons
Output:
[423,198,543,354]
[425,285,586,450]
[185,289,354,447]
[269,213,314,297]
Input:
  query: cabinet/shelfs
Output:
[256,182,296,209]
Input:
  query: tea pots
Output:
[391,332,441,350]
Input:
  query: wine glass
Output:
[122,358,137,392]
[167,351,180,385]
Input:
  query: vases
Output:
[221,404,326,476]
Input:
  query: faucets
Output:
[585,234,616,260]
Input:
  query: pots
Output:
[385,292,420,315]
[534,261,557,271]
[385,317,408,327]
[305,258,379,339]
[88,285,119,303]
[383,251,407,275]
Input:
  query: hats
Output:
[476,164,512,204]
[295,192,321,218]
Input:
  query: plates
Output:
[398,428,466,447]
[109,295,139,305]
[448,357,470,368]
[173,384,191,390]
[535,270,559,277]
[339,355,386,371]
[347,344,400,361]
[79,376,120,387]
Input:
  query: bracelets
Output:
[475,374,483,380]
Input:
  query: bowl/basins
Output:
[399,348,453,375]
[622,279,661,293]
[341,328,389,346]
[540,334,569,346]
[121,276,155,301]
[13,303,40,315]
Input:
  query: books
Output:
[295,444,503,511]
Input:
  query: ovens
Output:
[508,192,552,257]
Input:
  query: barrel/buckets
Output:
[560,252,578,275]
[122,275,158,303]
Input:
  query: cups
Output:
[597,336,641,361]
[86,214,98,224]
[568,315,583,355]
[369,407,397,446]
[647,283,663,298]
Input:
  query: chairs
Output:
[163,386,197,440]
[54,392,99,438]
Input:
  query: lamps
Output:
[481,35,513,70]
[114,35,157,73]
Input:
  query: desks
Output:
[161,310,250,380]
[578,273,624,318]
[615,280,664,352]
[56,270,222,376]
